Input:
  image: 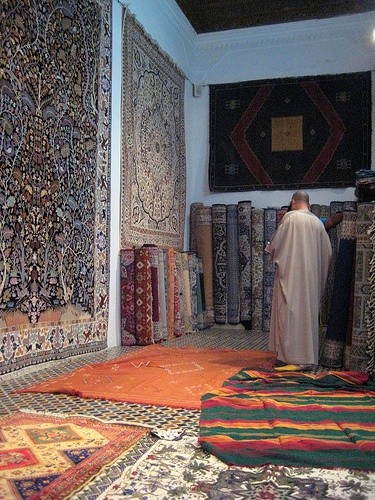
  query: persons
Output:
[266,190,332,370]
[286,200,343,235]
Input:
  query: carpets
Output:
[0,0,112,385]
[119,8,189,251]
[10,343,278,411]
[0,409,375,500]
